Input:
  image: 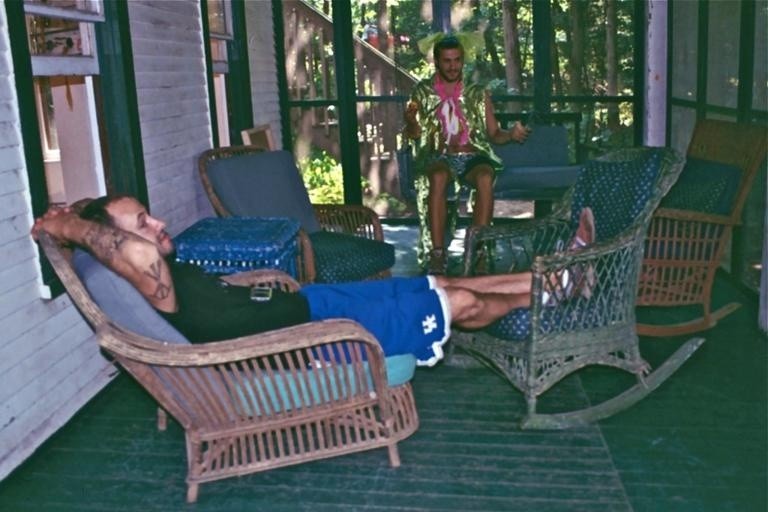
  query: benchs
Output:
[399,112,586,221]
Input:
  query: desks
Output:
[171,217,302,284]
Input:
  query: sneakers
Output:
[572,206,594,296]
[428,248,448,274]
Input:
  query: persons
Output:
[404,38,533,277]
[30,192,597,369]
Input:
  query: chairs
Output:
[444,147,706,431]
[199,145,394,279]
[633,111,768,337]
[38,229,419,503]
